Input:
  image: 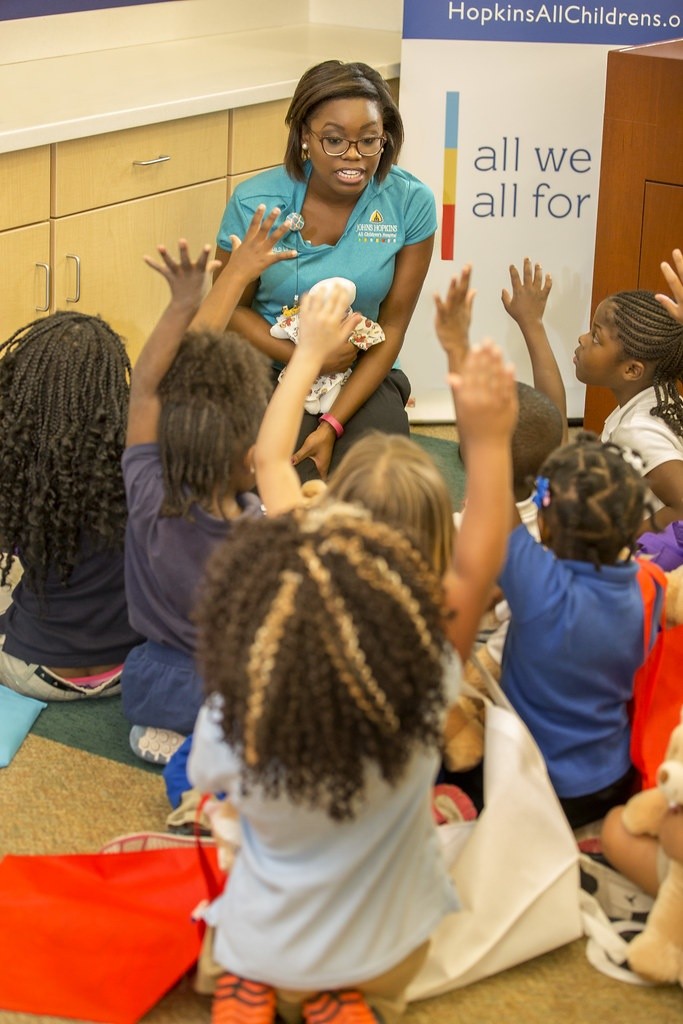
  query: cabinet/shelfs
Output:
[0,110,229,386]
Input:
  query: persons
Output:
[213,61,437,485]
[0,205,683,1024]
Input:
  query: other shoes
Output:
[575,819,606,854]
[98,822,215,853]
[302,988,379,1024]
[211,973,277,1024]
[431,784,471,826]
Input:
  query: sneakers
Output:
[130,725,186,765]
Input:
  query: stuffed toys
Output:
[270,277,386,415]
[622,722,683,988]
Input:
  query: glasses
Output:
[302,120,386,157]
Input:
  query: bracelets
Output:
[318,413,344,438]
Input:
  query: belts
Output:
[25,661,121,695]
